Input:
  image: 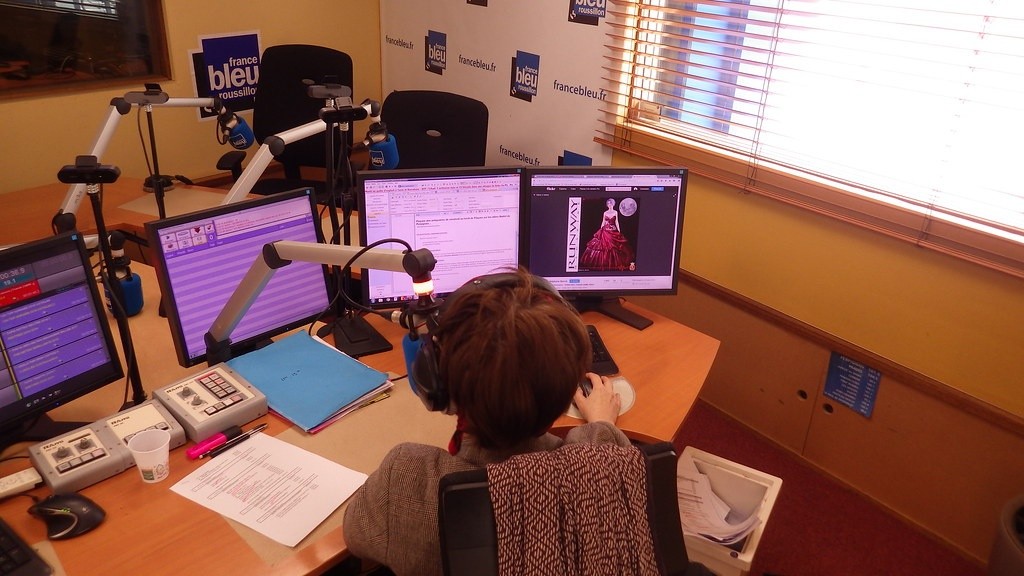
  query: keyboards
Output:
[584,325,620,376]
[0,517,52,576]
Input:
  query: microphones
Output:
[217,111,254,149]
[380,309,441,340]
[366,122,400,170]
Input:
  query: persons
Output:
[106,32,153,77]
[580,199,635,271]
[343,264,633,576]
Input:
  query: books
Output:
[224,329,394,434]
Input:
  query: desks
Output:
[0,175,721,575]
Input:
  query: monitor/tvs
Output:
[0,230,125,452]
[143,187,336,368]
[356,166,689,331]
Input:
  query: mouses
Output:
[27,492,106,540]
[573,378,593,408]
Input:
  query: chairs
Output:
[216,44,489,210]
[437,442,712,575]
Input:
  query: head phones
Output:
[410,274,580,413]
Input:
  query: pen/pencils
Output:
[199,422,268,459]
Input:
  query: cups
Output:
[128,429,170,483]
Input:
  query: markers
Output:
[186,425,242,460]
